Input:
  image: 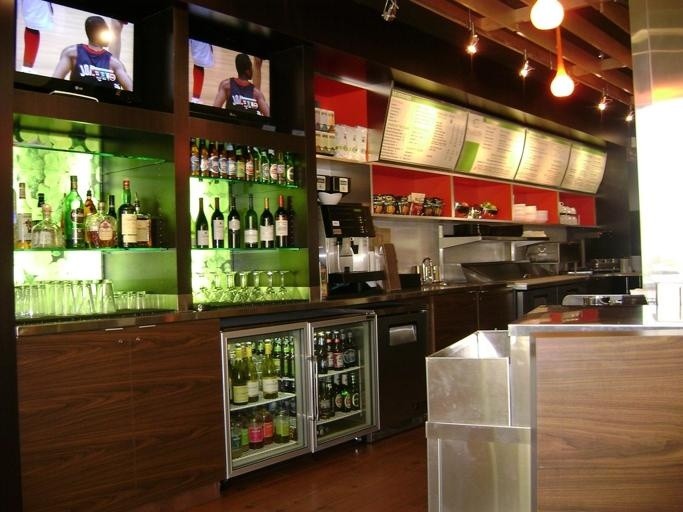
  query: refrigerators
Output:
[219,310,380,482]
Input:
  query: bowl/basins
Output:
[514,202,547,222]
[318,191,342,205]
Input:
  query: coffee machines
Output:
[319,203,386,300]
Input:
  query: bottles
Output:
[12,175,151,250]
[244,193,258,248]
[211,198,224,247]
[374,195,443,216]
[559,201,578,225]
[259,197,274,247]
[189,137,296,187]
[274,194,288,248]
[226,327,358,405]
[227,197,240,248]
[194,197,208,249]
[230,372,360,459]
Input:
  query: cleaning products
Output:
[433,264,440,282]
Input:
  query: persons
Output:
[213,54,270,117]
[252,56,262,91]
[16,0,53,68]
[53,16,133,92]
[189,39,214,105]
[109,18,128,60]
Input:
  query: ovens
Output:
[515,281,593,320]
[367,308,433,447]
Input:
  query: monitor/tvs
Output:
[188,36,280,131]
[14,0,146,106]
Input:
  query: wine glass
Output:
[193,271,291,301]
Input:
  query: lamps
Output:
[382,0,633,122]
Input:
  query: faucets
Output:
[421,257,433,285]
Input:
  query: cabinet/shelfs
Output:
[12,320,220,504]
[0,0,320,333]
[369,161,597,228]
[516,274,590,313]
[430,282,516,353]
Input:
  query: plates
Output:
[453,223,523,237]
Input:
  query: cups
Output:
[616,256,641,272]
[15,279,169,317]
